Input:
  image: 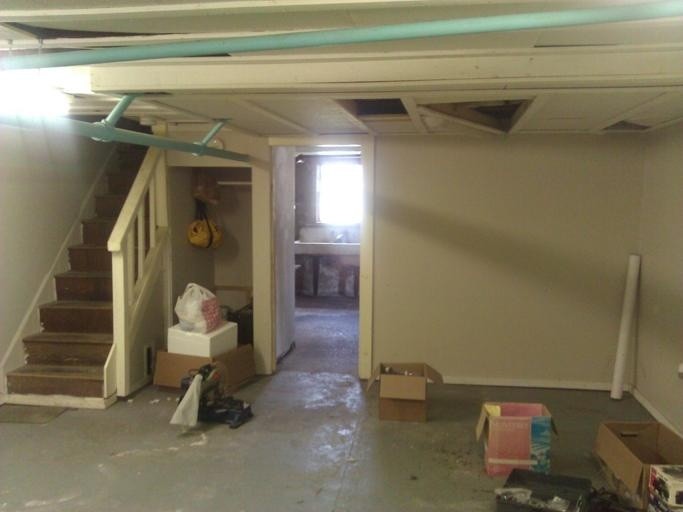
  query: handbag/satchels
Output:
[187,219,224,249]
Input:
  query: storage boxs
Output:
[646,463,683,511]
[475,401,560,476]
[152,343,257,396]
[167,320,238,357]
[591,420,683,512]
[366,363,443,422]
